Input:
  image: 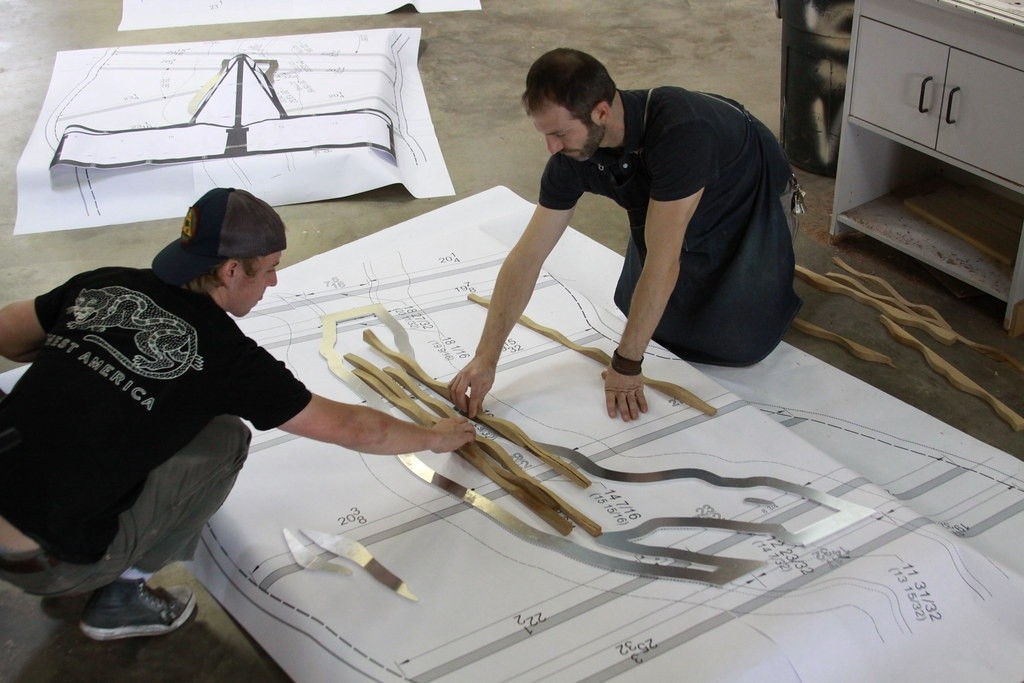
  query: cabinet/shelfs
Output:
[826,0,1024,340]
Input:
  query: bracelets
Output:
[612,350,644,375]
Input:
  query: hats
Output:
[153,187,289,288]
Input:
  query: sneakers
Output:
[80,575,203,640]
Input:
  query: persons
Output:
[0,187,476,640]
[448,48,804,422]
